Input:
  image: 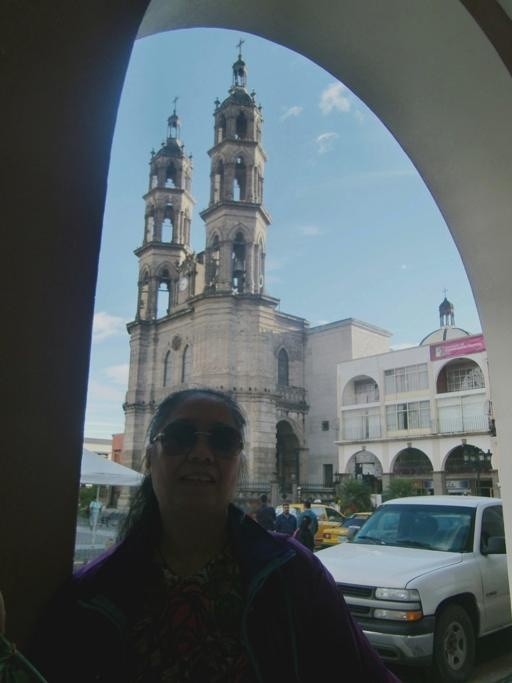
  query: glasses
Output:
[150,417,245,462]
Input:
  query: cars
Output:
[323,511,376,545]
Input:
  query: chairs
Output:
[404,516,439,546]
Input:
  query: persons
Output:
[24,384,401,682]
[256,492,318,554]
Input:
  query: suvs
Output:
[274,502,345,547]
[307,492,511,682]
